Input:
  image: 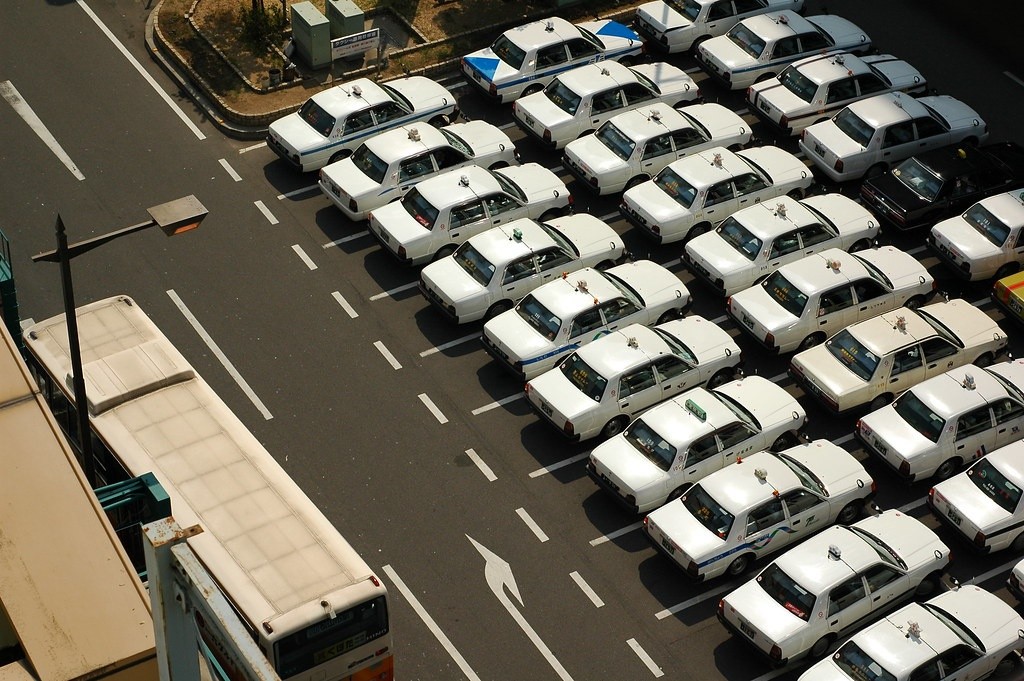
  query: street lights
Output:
[31,194,207,493]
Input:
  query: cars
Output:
[725,246,939,355]
[797,583,1024,681]
[586,374,810,517]
[619,144,816,244]
[858,140,1024,238]
[461,0,992,200]
[416,211,635,329]
[926,437,1024,557]
[642,438,876,584]
[716,507,953,666]
[479,259,693,385]
[925,187,1024,288]
[854,357,1024,484]
[680,193,882,304]
[526,314,745,446]
[317,120,522,228]
[363,162,576,273]
[265,74,461,182]
[787,298,1009,418]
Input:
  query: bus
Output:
[21,294,395,681]
